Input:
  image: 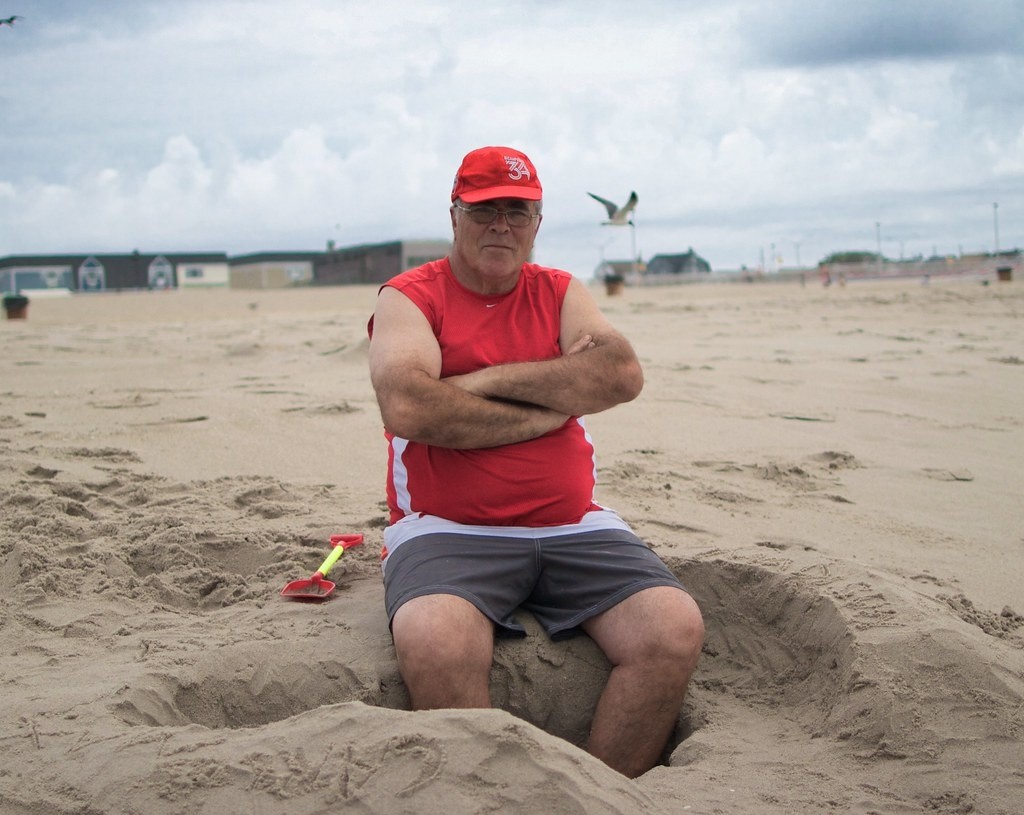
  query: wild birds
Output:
[0,15,27,27]
[587,190,639,227]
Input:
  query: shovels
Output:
[280,533,363,599]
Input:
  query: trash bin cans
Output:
[5,295,29,318]
[605,274,624,295]
[997,265,1011,280]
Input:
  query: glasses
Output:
[456,204,540,227]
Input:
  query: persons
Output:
[367,145,706,781]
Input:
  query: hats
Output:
[451,147,543,203]
